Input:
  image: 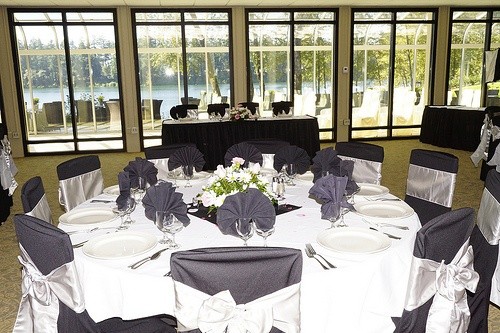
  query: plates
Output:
[352,200,415,220]
[83,230,156,259]
[351,181,390,199]
[318,225,390,254]
[168,170,210,183]
[58,207,117,227]
[103,183,119,198]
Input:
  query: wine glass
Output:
[115,162,357,249]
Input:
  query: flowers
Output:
[199,158,273,206]
[230,105,248,120]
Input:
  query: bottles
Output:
[272,177,278,192]
[275,179,285,195]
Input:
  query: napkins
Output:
[123,156,157,188]
[309,175,347,220]
[168,146,205,174]
[217,189,276,236]
[143,182,190,228]
[273,147,310,175]
[339,161,359,195]
[225,143,262,168]
[115,172,134,209]
[311,146,341,182]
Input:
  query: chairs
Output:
[171,102,294,121]
[36,100,162,131]
[278,87,484,127]
[13,139,500,333]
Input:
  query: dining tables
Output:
[420,105,486,152]
[162,115,320,159]
[57,170,422,333]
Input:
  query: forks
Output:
[304,244,335,271]
